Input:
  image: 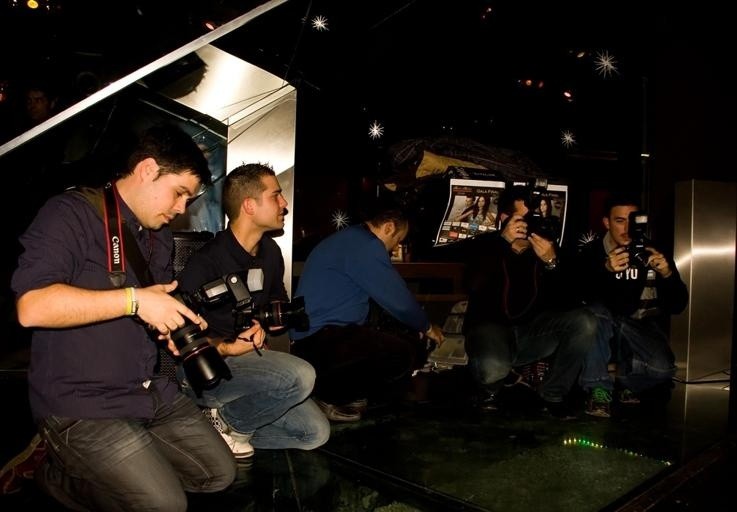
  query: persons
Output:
[447,185,612,420]
[535,198,552,216]
[460,194,475,223]
[175,164,331,458]
[456,193,496,229]
[10,123,237,511]
[547,193,688,419]
[288,197,444,422]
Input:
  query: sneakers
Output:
[477,390,499,412]
[582,388,609,418]
[1,429,55,495]
[618,384,641,404]
[537,379,564,405]
[207,408,255,459]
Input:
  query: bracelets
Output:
[125,286,137,316]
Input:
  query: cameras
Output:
[154,273,253,399]
[515,177,562,244]
[231,295,310,336]
[616,212,653,272]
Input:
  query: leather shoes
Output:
[316,396,366,423]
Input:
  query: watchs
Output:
[544,257,559,269]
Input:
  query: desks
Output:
[391,259,513,404]
